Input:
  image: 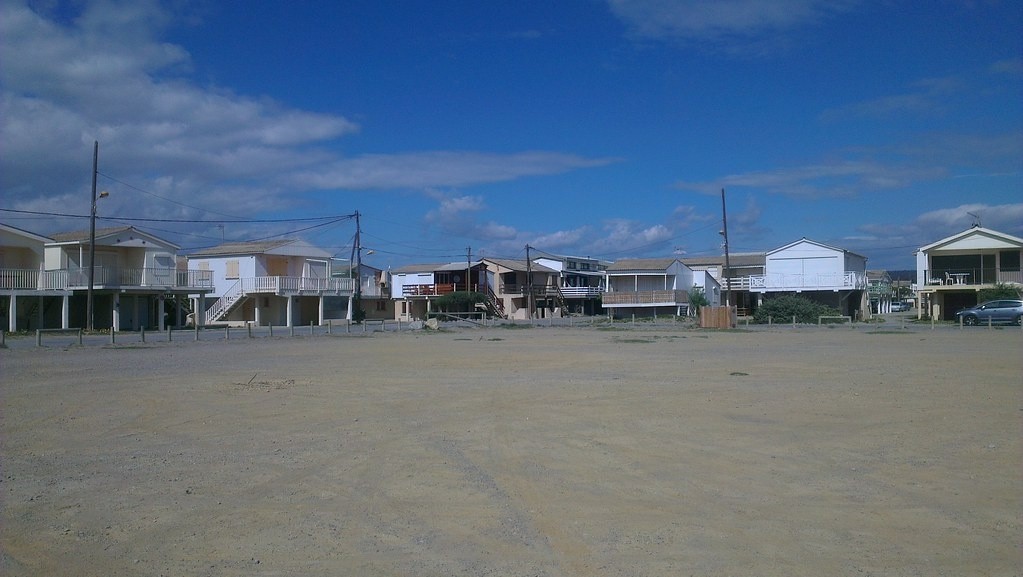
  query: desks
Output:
[950,273,970,285]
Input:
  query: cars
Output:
[954,300,1023,327]
[891,302,910,312]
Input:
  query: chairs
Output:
[945,272,953,285]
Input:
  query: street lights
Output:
[86,190,111,331]
[356,250,375,323]
[718,230,731,306]
[216,223,224,244]
